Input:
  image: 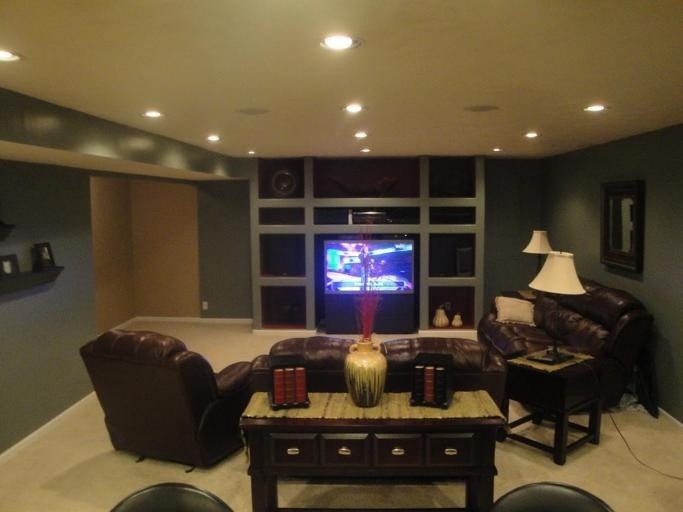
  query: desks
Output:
[239,390,508,512]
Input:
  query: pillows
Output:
[494,295,537,328]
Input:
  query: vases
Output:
[343,339,394,407]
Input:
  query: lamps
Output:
[521,230,552,300]
[526,250,586,365]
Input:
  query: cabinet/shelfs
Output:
[250,156,315,330]
[0,266,65,295]
[313,158,420,234]
[420,155,484,335]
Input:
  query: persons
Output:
[359,248,370,293]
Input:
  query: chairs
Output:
[110,481,233,512]
[493,481,611,512]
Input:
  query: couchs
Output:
[477,276,654,415]
[250,334,511,441]
[79,327,253,469]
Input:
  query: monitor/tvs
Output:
[323,239,415,295]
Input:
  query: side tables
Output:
[503,347,601,465]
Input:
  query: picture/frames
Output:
[599,180,643,273]
